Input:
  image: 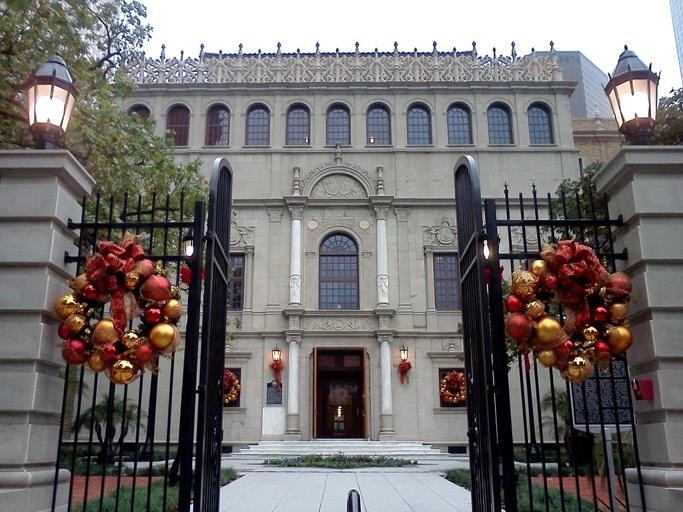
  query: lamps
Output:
[601,43,661,145]
[21,52,80,150]
